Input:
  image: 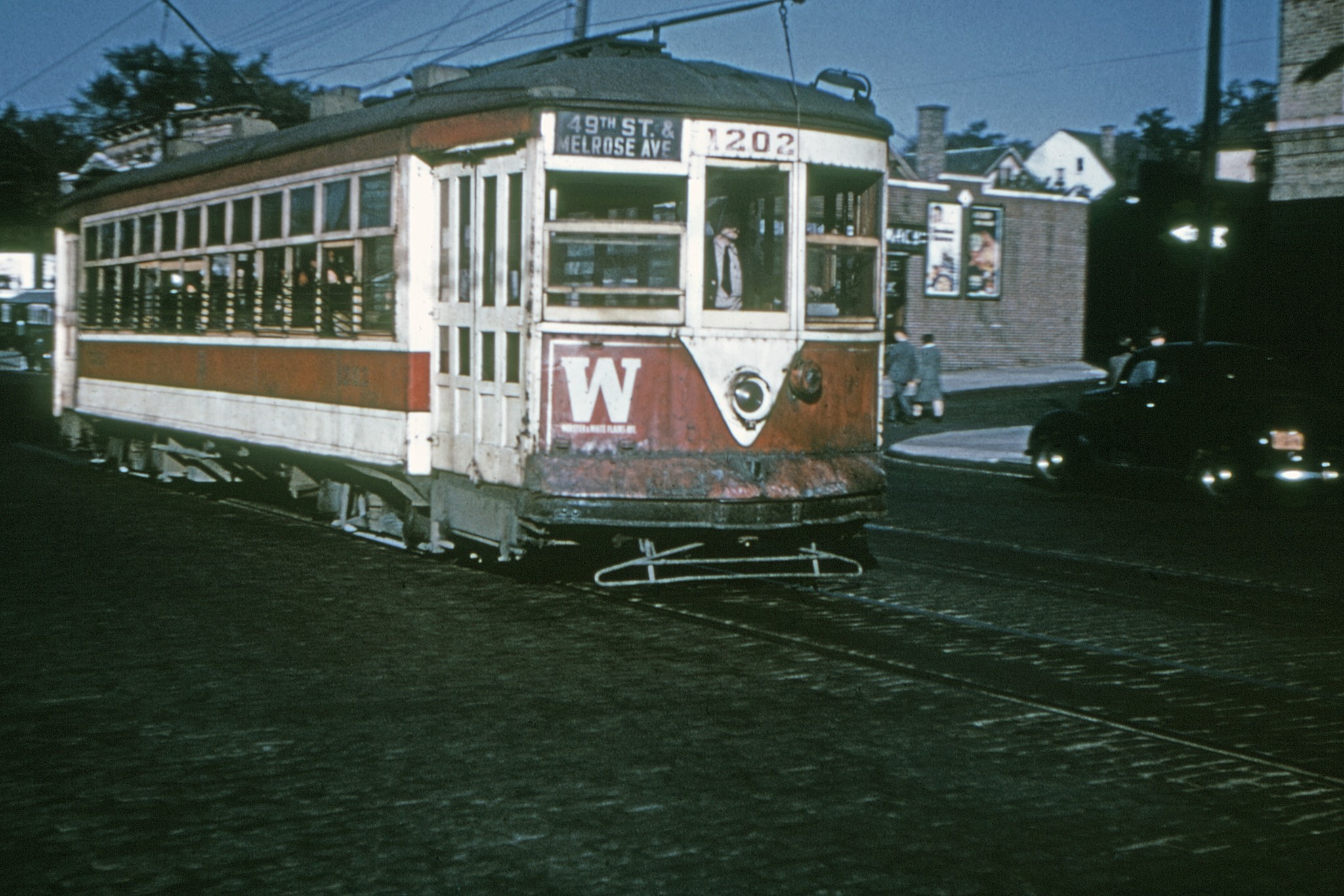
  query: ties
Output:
[728,245,742,296]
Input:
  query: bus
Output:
[37,48,900,595]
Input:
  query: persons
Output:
[913,331,945,422]
[883,325,918,423]
[968,230,1000,295]
[704,197,754,308]
